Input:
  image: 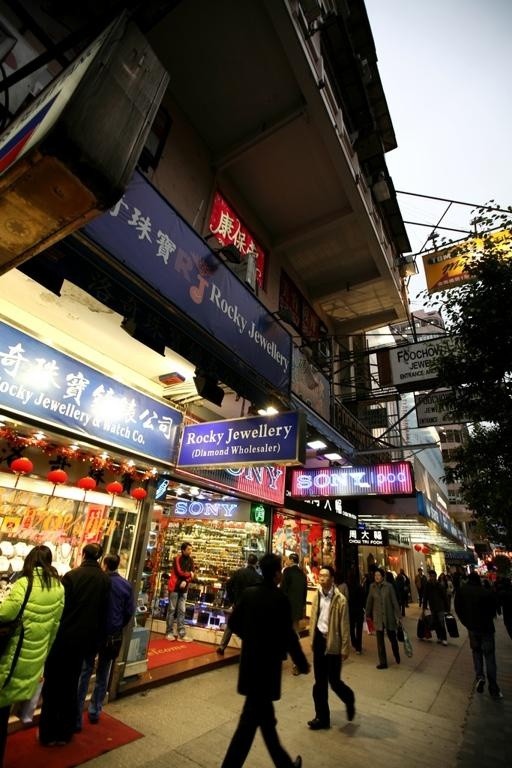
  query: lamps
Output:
[120,298,166,356]
[31,431,159,475]
[217,244,241,264]
[323,446,342,460]
[272,308,292,324]
[306,432,328,450]
[192,366,224,407]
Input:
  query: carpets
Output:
[147,635,216,671]
[2,705,144,768]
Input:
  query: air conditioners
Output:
[312,338,330,365]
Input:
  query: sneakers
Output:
[165,628,227,657]
[436,637,449,646]
[474,678,504,700]
[376,647,401,670]
[306,692,355,729]
[367,630,375,636]
[32,710,104,748]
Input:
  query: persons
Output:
[0,545,66,764]
[72,552,135,732]
[165,542,195,643]
[36,543,112,747]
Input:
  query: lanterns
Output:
[78,476,96,503]
[105,482,124,506]
[422,546,429,554]
[414,544,421,551]
[10,457,34,488]
[47,469,68,496]
[131,488,147,511]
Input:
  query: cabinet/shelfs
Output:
[151,598,242,649]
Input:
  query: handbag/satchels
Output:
[13,677,44,726]
[415,613,463,639]
[0,574,29,690]
[397,619,414,658]
[105,634,121,660]
[364,616,375,631]
